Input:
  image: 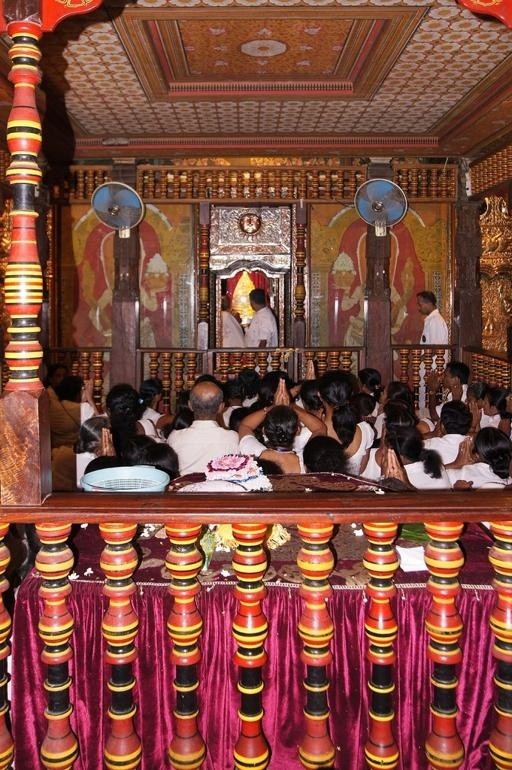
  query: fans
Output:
[90,183,145,239]
[353,178,407,237]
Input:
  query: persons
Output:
[302,369,511,489]
[47,368,328,493]
[412,291,447,409]
[220,298,246,348]
[239,288,278,374]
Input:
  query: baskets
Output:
[80,465,170,493]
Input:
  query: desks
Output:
[21,477,512,770]
